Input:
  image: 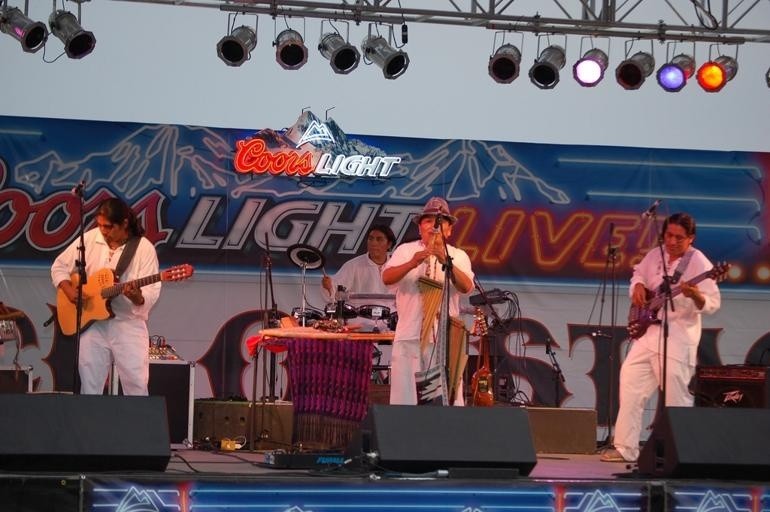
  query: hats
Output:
[412,196,458,225]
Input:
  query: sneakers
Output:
[600,448,625,462]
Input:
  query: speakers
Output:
[637,406,770,479]
[194,397,295,451]
[525,405,597,456]
[0,392,172,473]
[344,403,538,478]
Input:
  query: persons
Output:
[51,197,162,396]
[380,197,475,405]
[320,223,400,333]
[600,213,721,463]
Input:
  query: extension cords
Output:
[218,438,237,451]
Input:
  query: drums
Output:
[325,303,357,318]
[292,307,325,320]
[360,305,390,318]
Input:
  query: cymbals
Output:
[286,243,325,270]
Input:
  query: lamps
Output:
[48,0,96,60]
[485,23,745,95]
[0,0,49,54]
[216,0,411,82]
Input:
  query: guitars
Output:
[627,261,731,338]
[471,305,495,407]
[57,264,193,336]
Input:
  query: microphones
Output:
[69,178,86,195]
[432,207,442,233]
[268,301,280,327]
[641,198,662,220]
